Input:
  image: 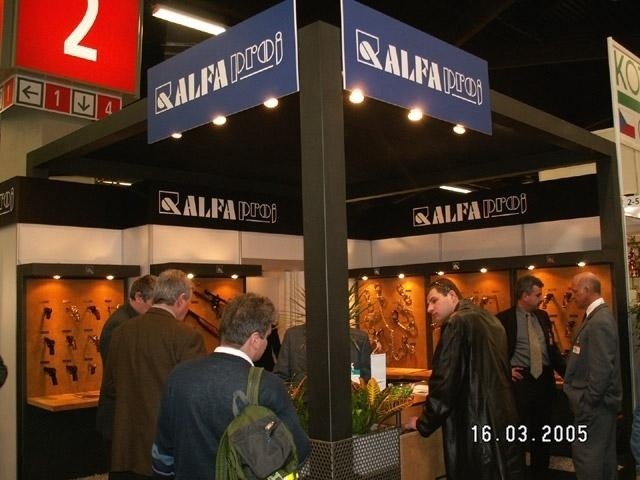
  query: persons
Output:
[101,269,208,480]
[150,292,314,480]
[94,274,158,473]
[495,275,567,480]
[274,317,372,383]
[254,323,281,372]
[563,270,623,480]
[403,279,527,480]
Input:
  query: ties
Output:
[526,312,543,380]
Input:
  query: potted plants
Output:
[283,371,416,479]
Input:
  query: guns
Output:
[192,289,228,319]
[186,309,221,338]
[43,303,118,385]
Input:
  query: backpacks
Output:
[214,365,302,480]
